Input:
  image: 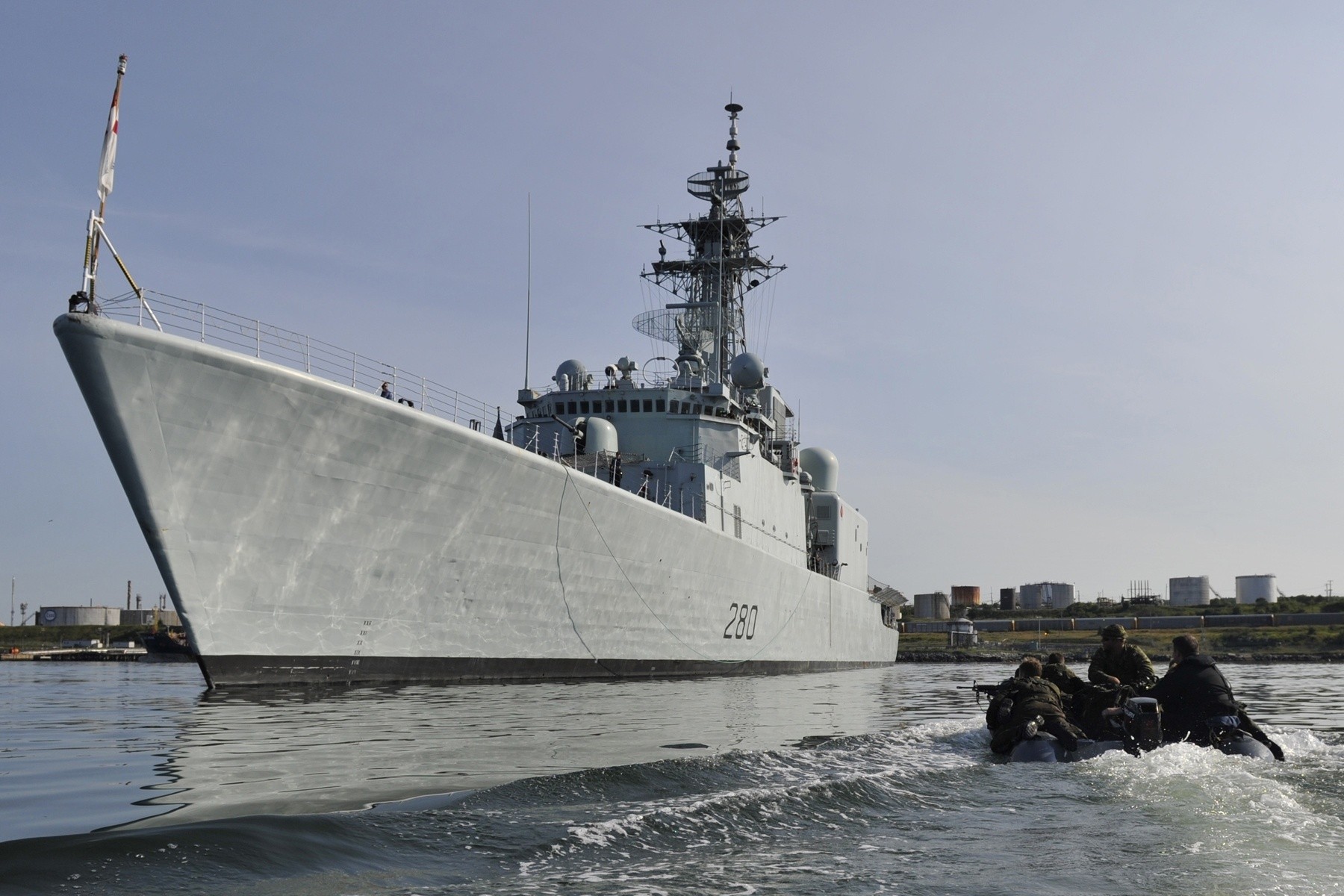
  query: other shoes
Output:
[1019,714,1045,740]
[1052,722,1077,751]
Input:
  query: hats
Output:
[1100,623,1128,638]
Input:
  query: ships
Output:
[48,97,908,688]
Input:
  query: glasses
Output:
[1103,637,1122,641]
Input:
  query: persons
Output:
[381,382,399,402]
[986,623,1285,761]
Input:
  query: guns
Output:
[956,680,1001,696]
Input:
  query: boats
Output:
[1010,696,1276,764]
